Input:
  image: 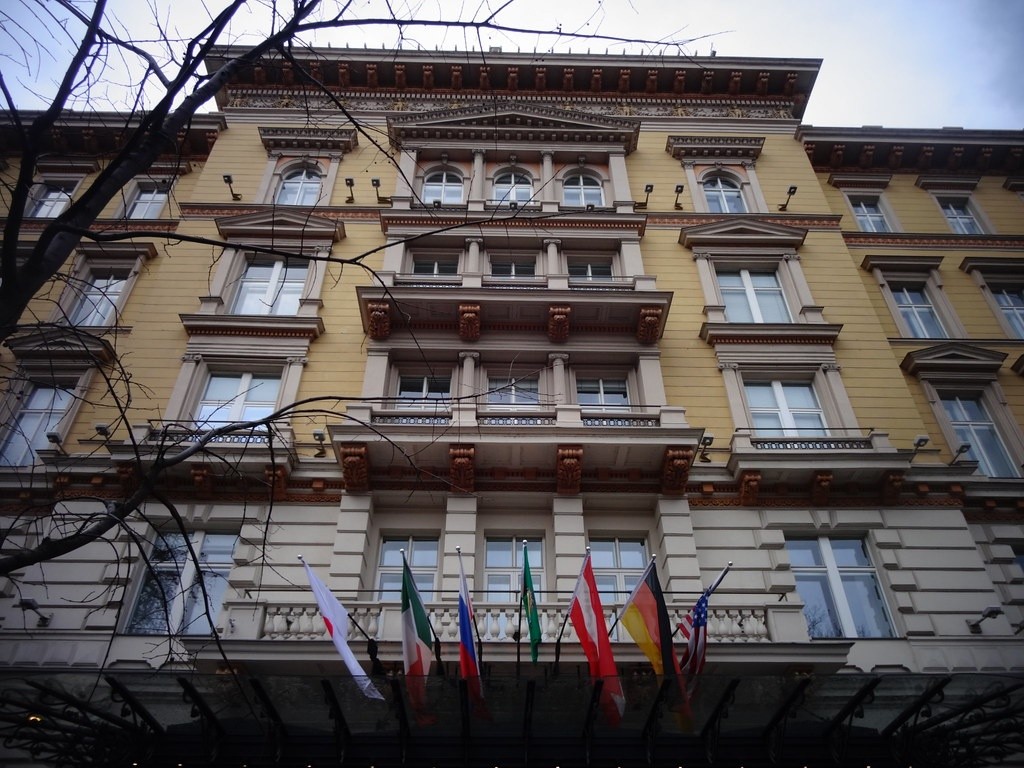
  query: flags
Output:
[402,558,435,727]
[679,590,709,700]
[619,564,695,727]
[303,561,385,699]
[448,587,482,724]
[521,546,542,666]
[568,556,625,730]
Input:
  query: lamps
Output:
[46,432,69,455]
[371,176,390,203]
[223,174,242,200]
[586,203,595,211]
[311,429,328,457]
[509,200,518,210]
[778,185,798,210]
[948,442,971,465]
[96,424,113,446]
[634,185,654,209]
[909,434,929,463]
[674,185,684,209]
[434,199,442,208]
[698,432,713,462]
[344,178,355,202]
[966,607,1001,634]
[21,597,51,626]
